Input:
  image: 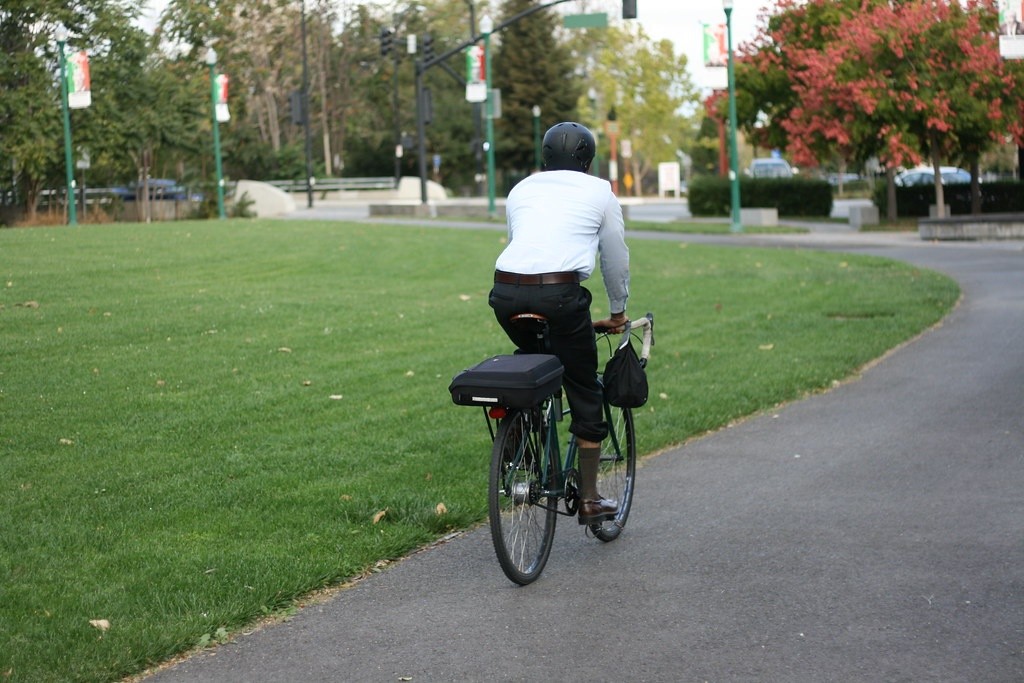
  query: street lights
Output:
[206,45,234,219]
[532,103,543,171]
[478,14,498,212]
[720,0,750,232]
[54,19,82,227]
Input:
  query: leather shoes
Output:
[577,494,620,525]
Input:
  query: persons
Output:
[488,122,630,525]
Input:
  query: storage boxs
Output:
[449,355,565,409]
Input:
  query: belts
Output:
[494,271,580,286]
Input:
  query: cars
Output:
[745,158,800,181]
[112,178,204,201]
[894,167,985,186]
[828,173,861,184]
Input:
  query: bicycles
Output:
[451,309,654,586]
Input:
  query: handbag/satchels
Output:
[603,321,649,410]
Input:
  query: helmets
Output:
[542,122,596,172]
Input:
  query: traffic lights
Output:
[285,92,302,124]
[423,37,434,63]
[381,29,394,56]
[424,86,434,126]
